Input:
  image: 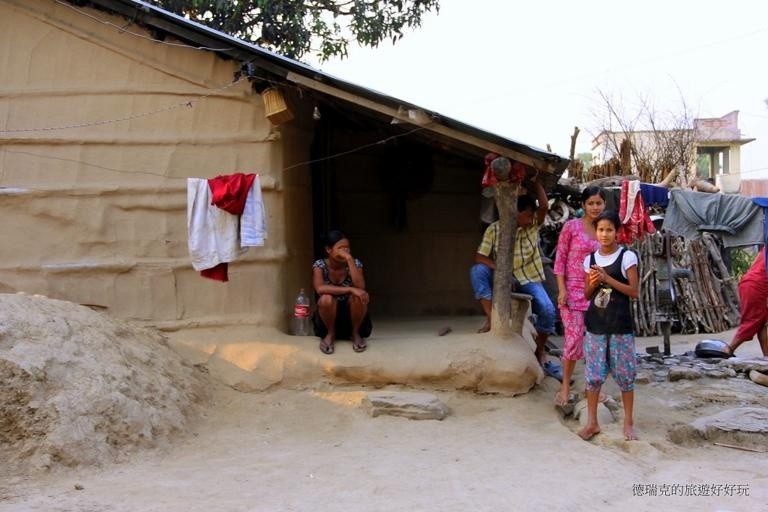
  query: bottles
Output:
[293,290,311,338]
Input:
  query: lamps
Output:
[312,102,321,120]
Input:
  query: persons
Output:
[312,231,373,354]
[469,177,556,368]
[576,212,642,441]
[730,246,768,357]
[554,185,607,407]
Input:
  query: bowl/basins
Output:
[693,338,731,358]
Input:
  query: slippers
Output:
[320,338,335,355]
[543,362,575,384]
[352,337,367,352]
